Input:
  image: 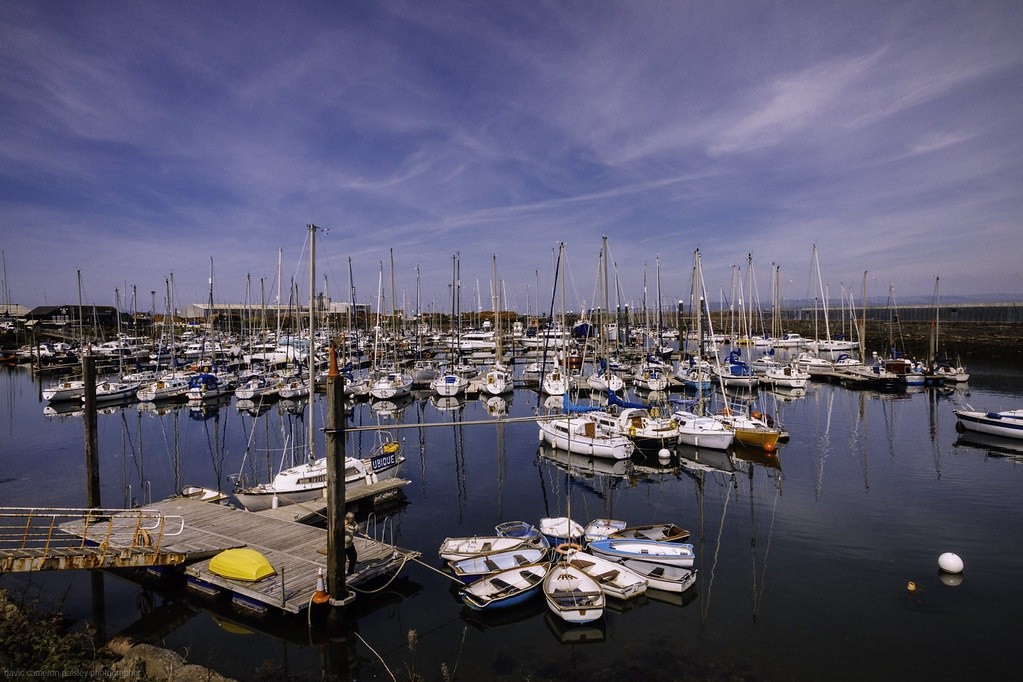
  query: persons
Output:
[345,511,363,576]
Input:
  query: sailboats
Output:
[0,219,974,626]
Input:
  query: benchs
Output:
[500,526,524,534]
[648,567,664,578]
[548,591,600,597]
[480,542,492,551]
[593,569,620,581]
[525,536,541,541]
[513,555,530,565]
[519,570,543,585]
[484,560,500,571]
[633,532,652,540]
[490,577,521,595]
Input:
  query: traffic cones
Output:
[312,567,331,604]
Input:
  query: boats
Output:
[643,580,699,608]
[207,549,277,583]
[951,401,1023,443]
[599,591,651,614]
[543,609,608,643]
[952,427,1023,461]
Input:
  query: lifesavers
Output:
[132,531,152,545]
[670,420,676,428]
[628,427,635,435]
[650,407,658,417]
[690,372,696,378]
[488,377,493,382]
[644,372,649,379]
[523,348,527,352]
[384,440,399,453]
[555,544,582,554]
[277,383,282,388]
